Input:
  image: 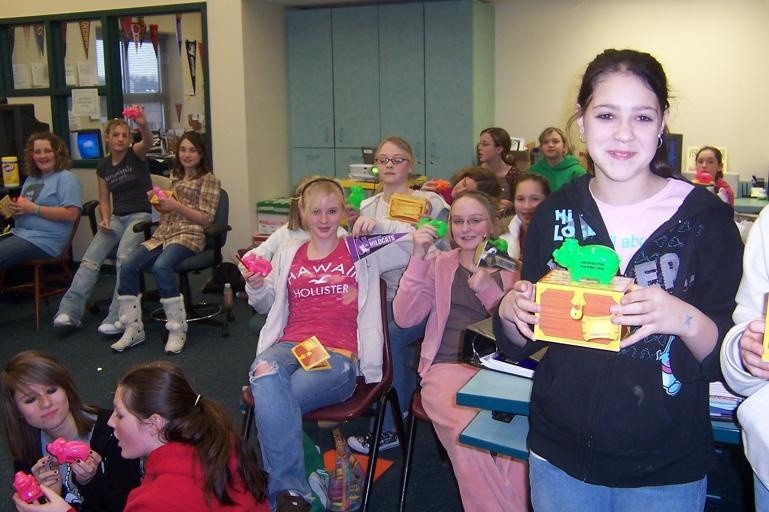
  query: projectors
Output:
[348,163,379,183]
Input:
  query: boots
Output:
[111,293,145,352]
[160,293,188,354]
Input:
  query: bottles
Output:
[223,283,234,310]
[236,292,249,299]
[1,156,20,187]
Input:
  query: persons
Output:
[721,205,769,511]
[111,131,222,355]
[1,132,83,284]
[494,46,745,511]
[11,362,274,511]
[686,145,734,207]
[53,105,153,334]
[236,175,457,512]
[1,348,140,511]
[341,125,586,511]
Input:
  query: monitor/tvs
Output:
[661,133,683,173]
[70,129,105,161]
[362,147,376,164]
[0,103,50,189]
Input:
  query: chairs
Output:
[133,188,232,343]
[242,280,408,511]
[0,210,92,332]
[398,391,446,511]
[80,200,160,314]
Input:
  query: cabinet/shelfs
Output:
[289,1,496,198]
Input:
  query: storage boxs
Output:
[257,198,291,234]
[505,142,535,170]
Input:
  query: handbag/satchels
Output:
[327,431,364,512]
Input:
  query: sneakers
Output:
[276,490,311,512]
[54,314,80,327]
[98,324,122,334]
[347,432,400,454]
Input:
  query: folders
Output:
[460,316,550,377]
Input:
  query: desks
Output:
[733,196,769,214]
[456,367,741,463]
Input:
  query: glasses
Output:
[376,157,408,164]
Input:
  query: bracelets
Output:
[172,201,182,215]
[35,204,41,216]
